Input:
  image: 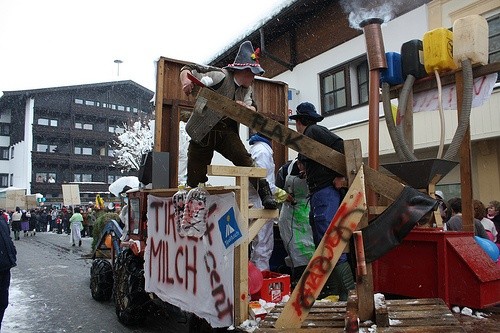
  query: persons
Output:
[278,158,316,289]
[0,215,24,331]
[428,189,500,250]
[11,206,22,240]
[69,208,84,247]
[240,128,288,271]
[288,103,357,301]
[2,204,125,240]
[179,42,280,211]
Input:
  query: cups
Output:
[271,283,282,302]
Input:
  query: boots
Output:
[252,178,277,210]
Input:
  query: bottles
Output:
[181,183,210,238]
[172,185,189,238]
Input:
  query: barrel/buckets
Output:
[378,52,404,88]
[452,14,489,66]
[401,39,427,80]
[422,27,458,76]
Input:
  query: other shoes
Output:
[338,291,349,302]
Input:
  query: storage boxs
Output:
[251,271,291,303]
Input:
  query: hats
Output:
[289,102,324,120]
[222,39,266,75]
